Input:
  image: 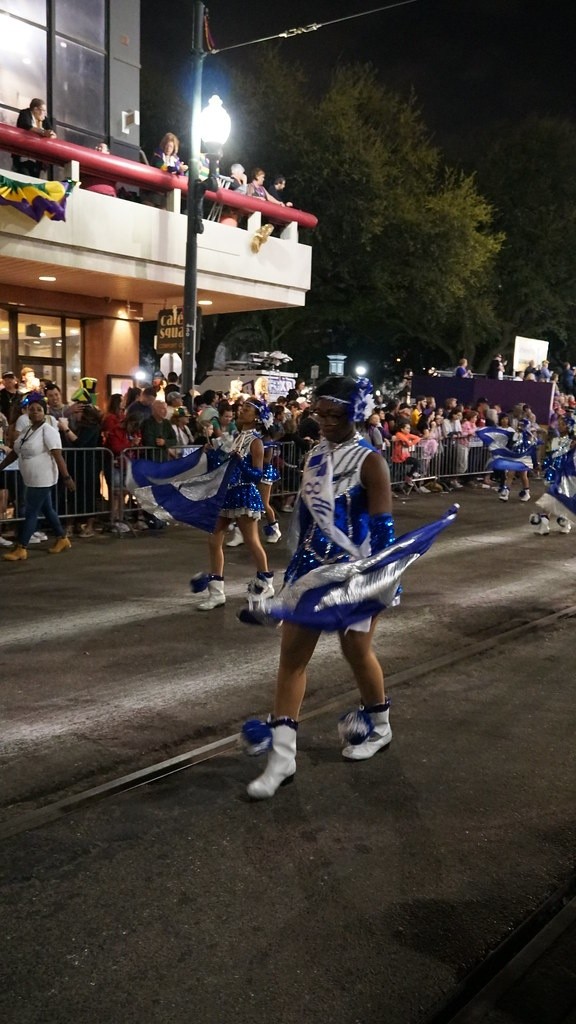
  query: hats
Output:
[166,392,186,402]
[421,408,433,417]
[413,396,426,404]
[398,403,412,410]
[565,406,575,414]
[2,370,15,379]
[173,406,191,417]
[476,397,493,404]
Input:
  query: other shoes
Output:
[390,468,543,498]
[0,500,169,562]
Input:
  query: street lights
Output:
[180,92,233,416]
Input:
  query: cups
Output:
[59,417,68,422]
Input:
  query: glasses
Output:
[308,410,340,426]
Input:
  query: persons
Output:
[535,418,576,536]
[197,355,576,512]
[0,368,196,560]
[143,134,189,206]
[195,398,275,611]
[225,428,282,546]
[12,99,57,177]
[244,374,395,800]
[270,178,293,207]
[248,167,284,207]
[84,142,116,197]
[220,164,248,226]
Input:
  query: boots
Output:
[529,513,550,536]
[236,712,299,800]
[518,489,531,503]
[336,694,393,761]
[263,521,283,544]
[225,524,245,547]
[189,571,227,611]
[498,486,510,502]
[243,573,275,603]
[556,516,572,534]
